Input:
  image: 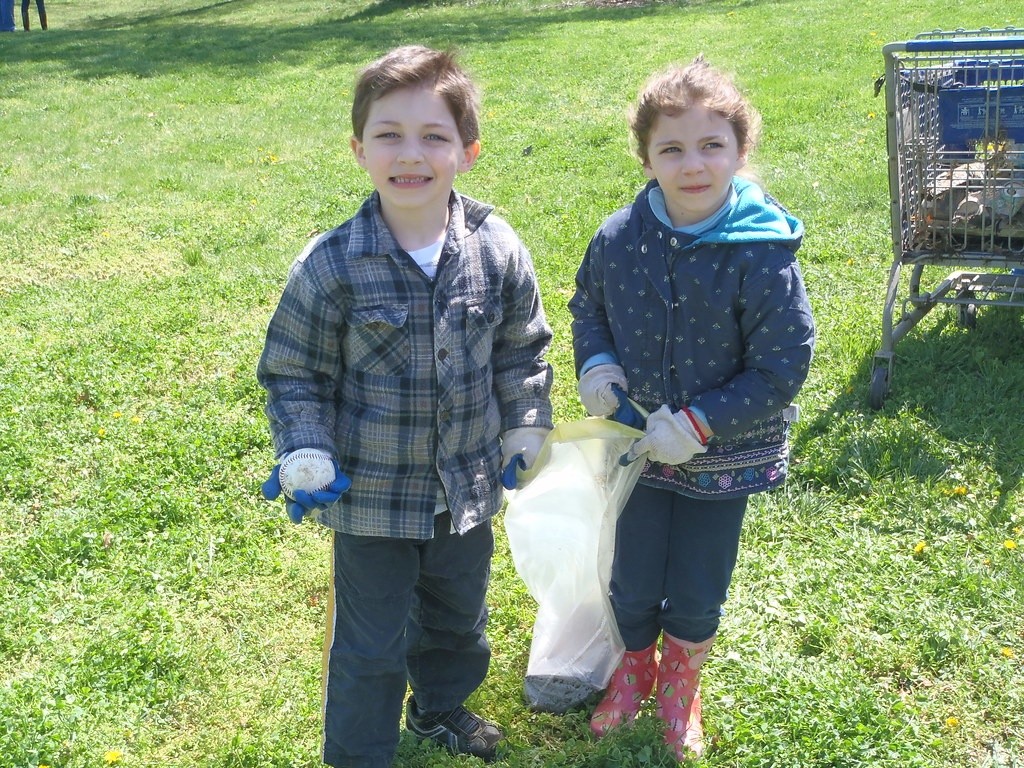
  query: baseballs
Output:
[278,446,336,499]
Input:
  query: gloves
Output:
[500,426,552,490]
[262,452,351,525]
[578,364,629,416]
[618,404,709,467]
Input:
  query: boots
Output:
[658,630,717,762]
[590,637,659,737]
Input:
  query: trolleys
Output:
[866,24,1024,410]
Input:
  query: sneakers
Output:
[404,692,508,760]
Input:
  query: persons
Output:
[565,52,816,764]
[20,0,47,33]
[256,44,553,768]
